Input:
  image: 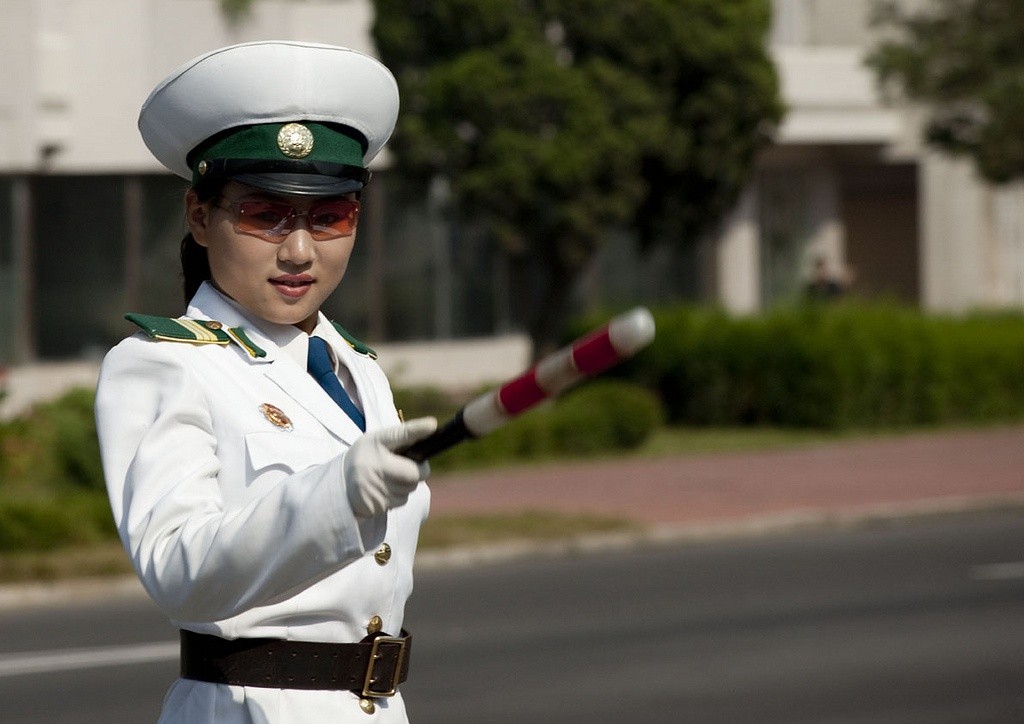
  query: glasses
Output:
[203,196,364,242]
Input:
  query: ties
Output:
[307,335,366,430]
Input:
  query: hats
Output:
[137,41,400,197]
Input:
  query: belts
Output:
[178,628,414,698]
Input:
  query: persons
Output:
[95,40,433,724]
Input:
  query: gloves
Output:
[344,414,445,516]
